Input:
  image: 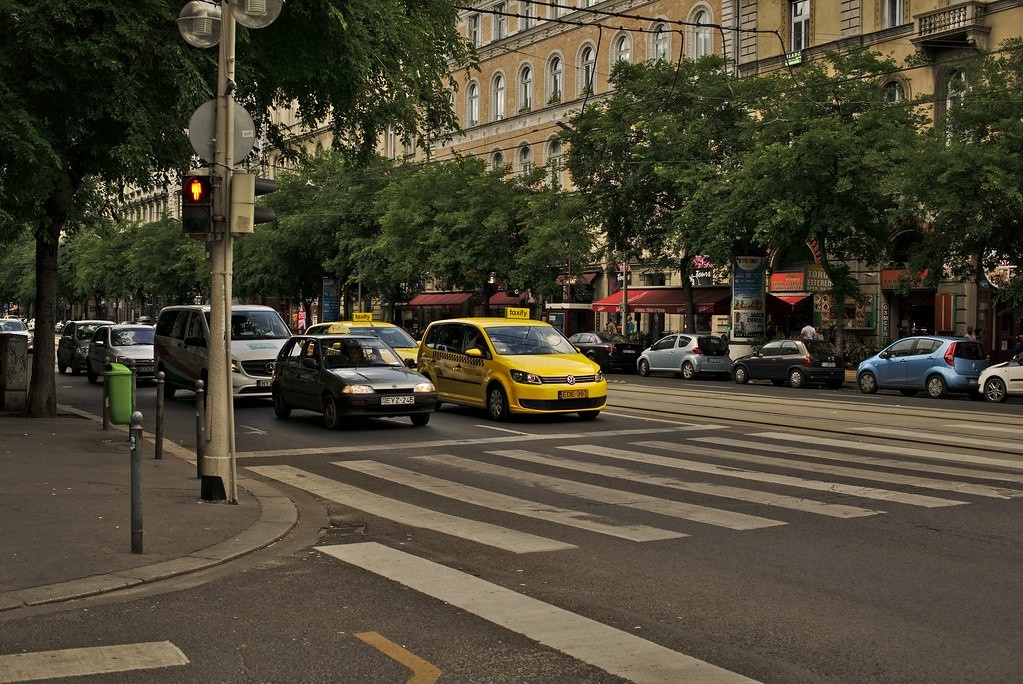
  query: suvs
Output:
[0,317,35,352]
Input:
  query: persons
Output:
[801,318,824,340]
[627,316,635,334]
[605,318,618,335]
[765,321,785,341]
[348,343,378,364]
[965,326,976,340]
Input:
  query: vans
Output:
[57,320,119,375]
[418,316,607,420]
[855,335,989,399]
[154,305,302,406]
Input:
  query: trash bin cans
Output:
[102,362,132,425]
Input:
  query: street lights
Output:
[175,0,286,505]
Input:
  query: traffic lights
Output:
[181,174,211,234]
[230,172,275,237]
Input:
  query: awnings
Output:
[484,284,532,310]
[554,272,597,286]
[403,289,477,312]
[593,284,734,315]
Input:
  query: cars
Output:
[302,322,422,372]
[732,339,844,388]
[5,314,35,331]
[271,333,437,430]
[86,324,159,384]
[977,351,1023,403]
[637,334,733,380]
[568,331,642,374]
